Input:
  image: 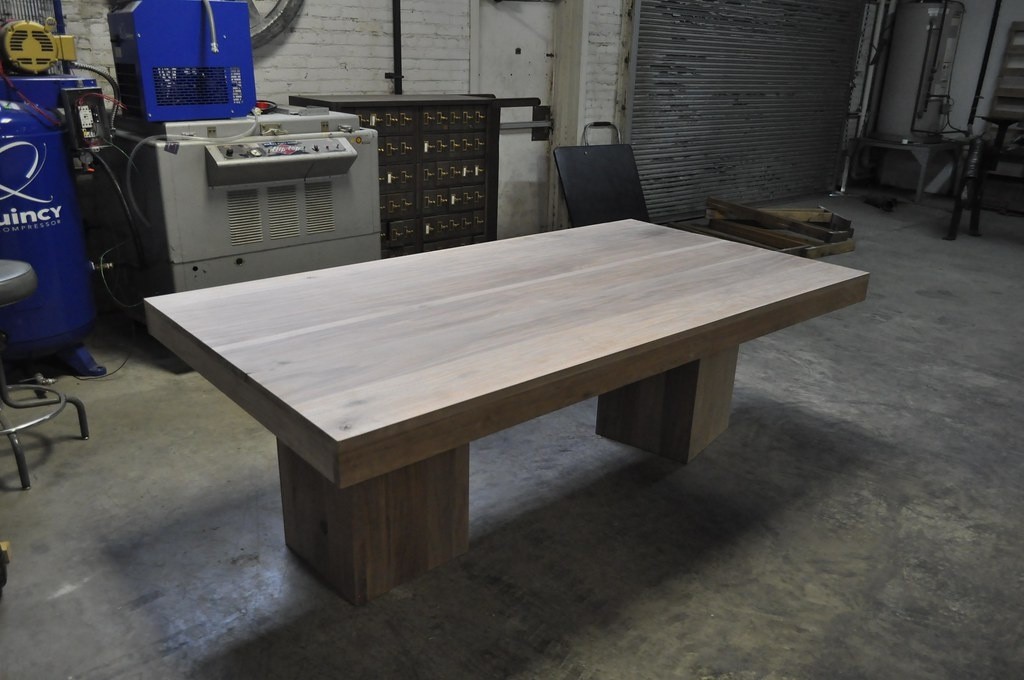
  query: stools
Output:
[0,258,89,489]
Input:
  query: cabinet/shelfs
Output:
[289,95,500,260]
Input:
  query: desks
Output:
[841,137,962,203]
[144,217,870,607]
